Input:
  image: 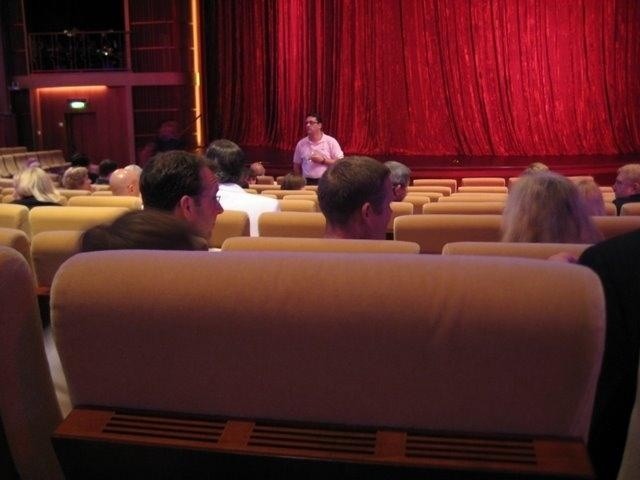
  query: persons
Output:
[9,168,62,211]
[94,159,117,186]
[293,114,344,186]
[244,162,265,185]
[138,151,224,252]
[316,156,393,240]
[523,163,549,176]
[63,167,96,193]
[575,179,605,217]
[612,164,640,216]
[499,173,602,244]
[138,122,186,169]
[204,139,280,238]
[70,152,98,185]
[548,229,640,480]
[78,209,209,253]
[385,161,411,202]
[110,169,140,198]
[280,174,307,190]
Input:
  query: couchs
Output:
[0,245,66,479]
[52,250,606,474]
[0,177,636,295]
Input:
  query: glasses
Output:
[197,195,222,204]
[305,120,318,124]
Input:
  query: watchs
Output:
[321,157,325,166]
